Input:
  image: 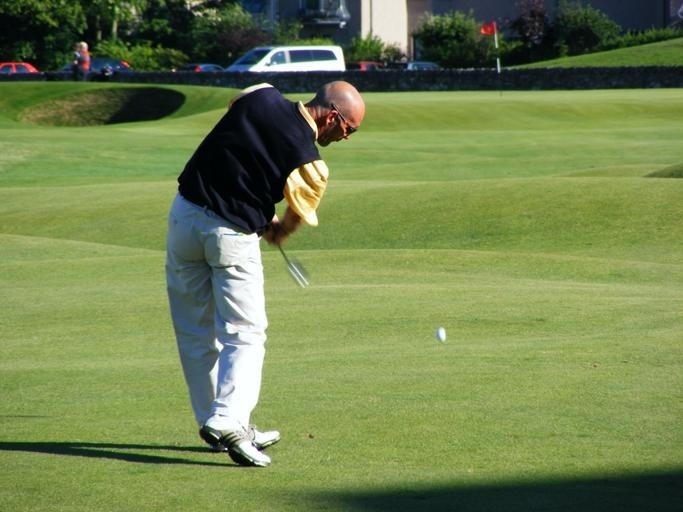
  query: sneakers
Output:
[245,426,282,449]
[199,415,273,468]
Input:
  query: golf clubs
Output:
[279,243,311,286]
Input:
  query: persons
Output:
[165,80,365,467]
[72,42,90,81]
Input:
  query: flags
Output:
[481,21,496,35]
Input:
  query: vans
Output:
[226,45,345,76]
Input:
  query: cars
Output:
[387,62,441,71]
[348,61,384,73]
[180,63,223,75]
[0,62,38,78]
[61,56,132,76]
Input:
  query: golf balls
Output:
[436,327,446,342]
